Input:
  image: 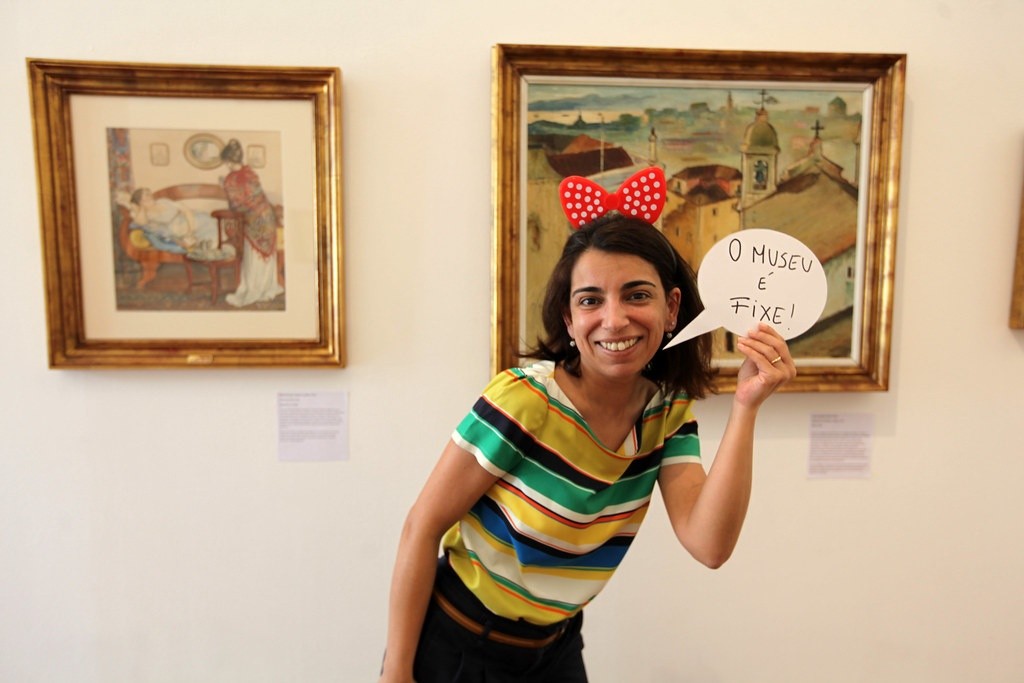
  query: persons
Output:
[374,217,803,683]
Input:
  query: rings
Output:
[769,357,784,368]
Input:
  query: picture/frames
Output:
[19,54,350,375]
[491,38,907,397]
